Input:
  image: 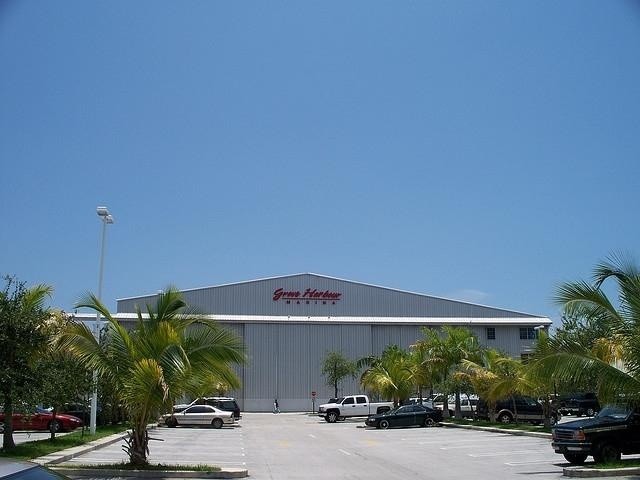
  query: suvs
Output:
[170,396,242,423]
[550,399,640,465]
[327,397,349,421]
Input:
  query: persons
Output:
[272,400,280,413]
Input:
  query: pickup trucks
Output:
[316,394,396,425]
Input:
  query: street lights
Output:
[88,203,118,439]
[533,323,546,349]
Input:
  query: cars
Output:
[159,403,238,429]
[0,455,74,480]
[1,394,125,434]
[363,401,444,429]
[408,391,602,425]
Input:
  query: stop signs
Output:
[312,391,316,396]
